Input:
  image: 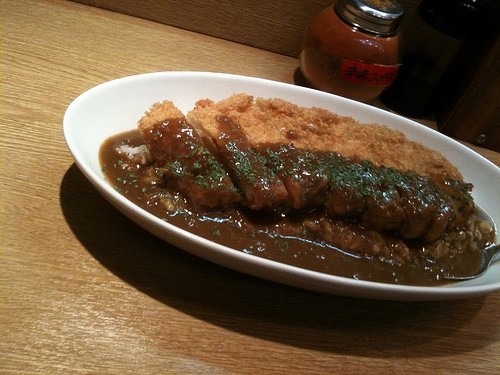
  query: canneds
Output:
[299,0,405,105]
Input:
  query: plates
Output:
[62,71,500,303]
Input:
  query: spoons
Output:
[417,243,500,280]
